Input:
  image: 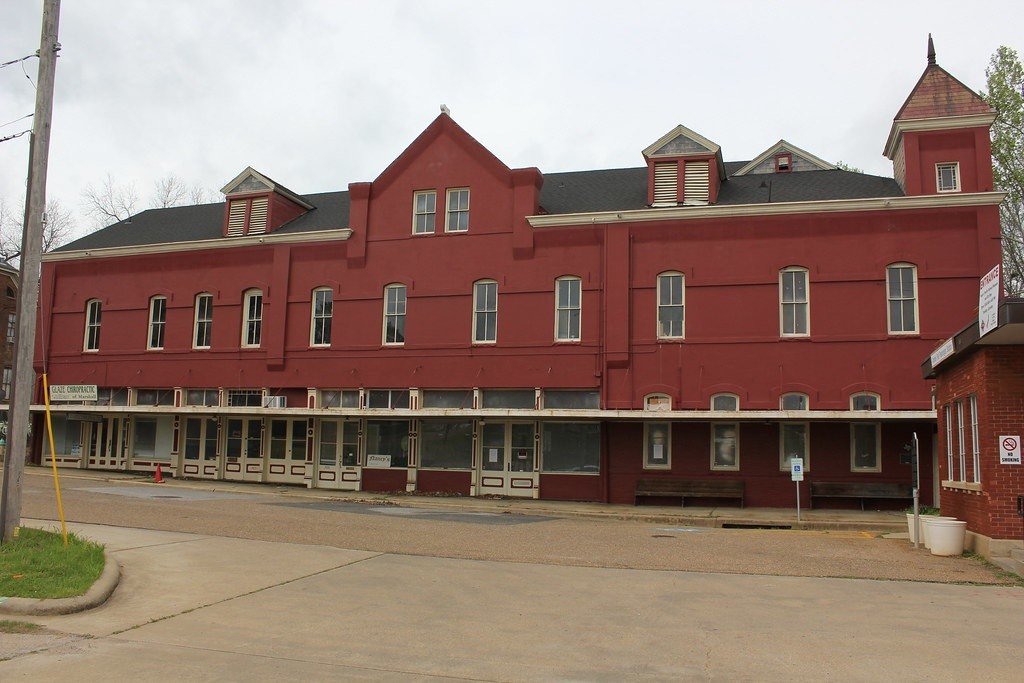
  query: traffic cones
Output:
[152,463,166,483]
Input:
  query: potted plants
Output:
[906,504,941,543]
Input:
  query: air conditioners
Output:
[262,396,287,407]
